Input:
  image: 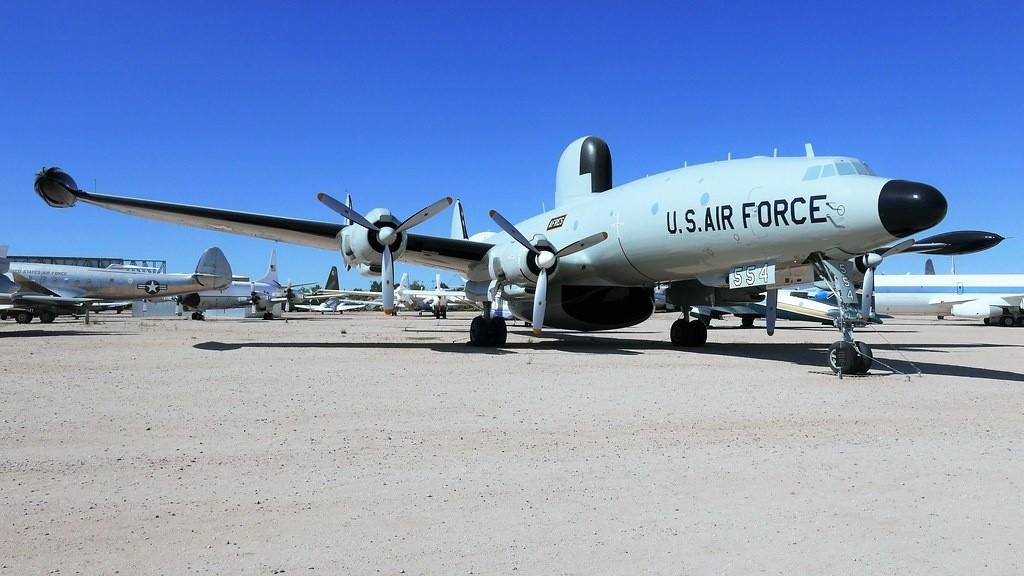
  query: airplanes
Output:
[1,134,1024,379]
